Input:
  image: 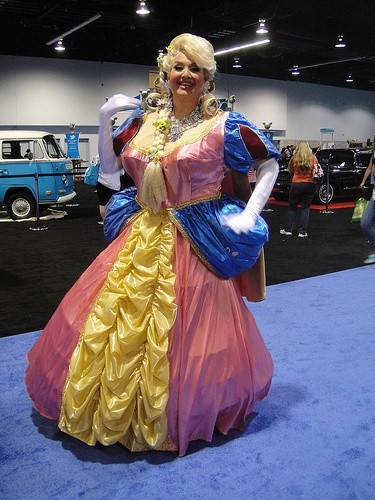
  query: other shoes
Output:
[363,254,375,263]
[280,229,292,235]
[298,233,308,237]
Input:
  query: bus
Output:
[1,129,77,220]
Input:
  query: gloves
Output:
[227,156,280,234]
[98,93,141,190]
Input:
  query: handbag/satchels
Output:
[312,156,324,178]
[351,198,370,222]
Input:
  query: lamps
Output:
[232,54,241,68]
[334,31,348,49]
[291,64,301,75]
[255,16,269,34]
[135,0,152,15]
[213,36,272,58]
[44,8,103,48]
[54,38,65,52]
[287,55,367,72]
[344,70,354,82]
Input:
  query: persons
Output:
[98,140,135,222]
[281,140,319,237]
[360,137,375,269]
[25,32,281,456]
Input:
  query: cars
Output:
[273,147,375,204]
